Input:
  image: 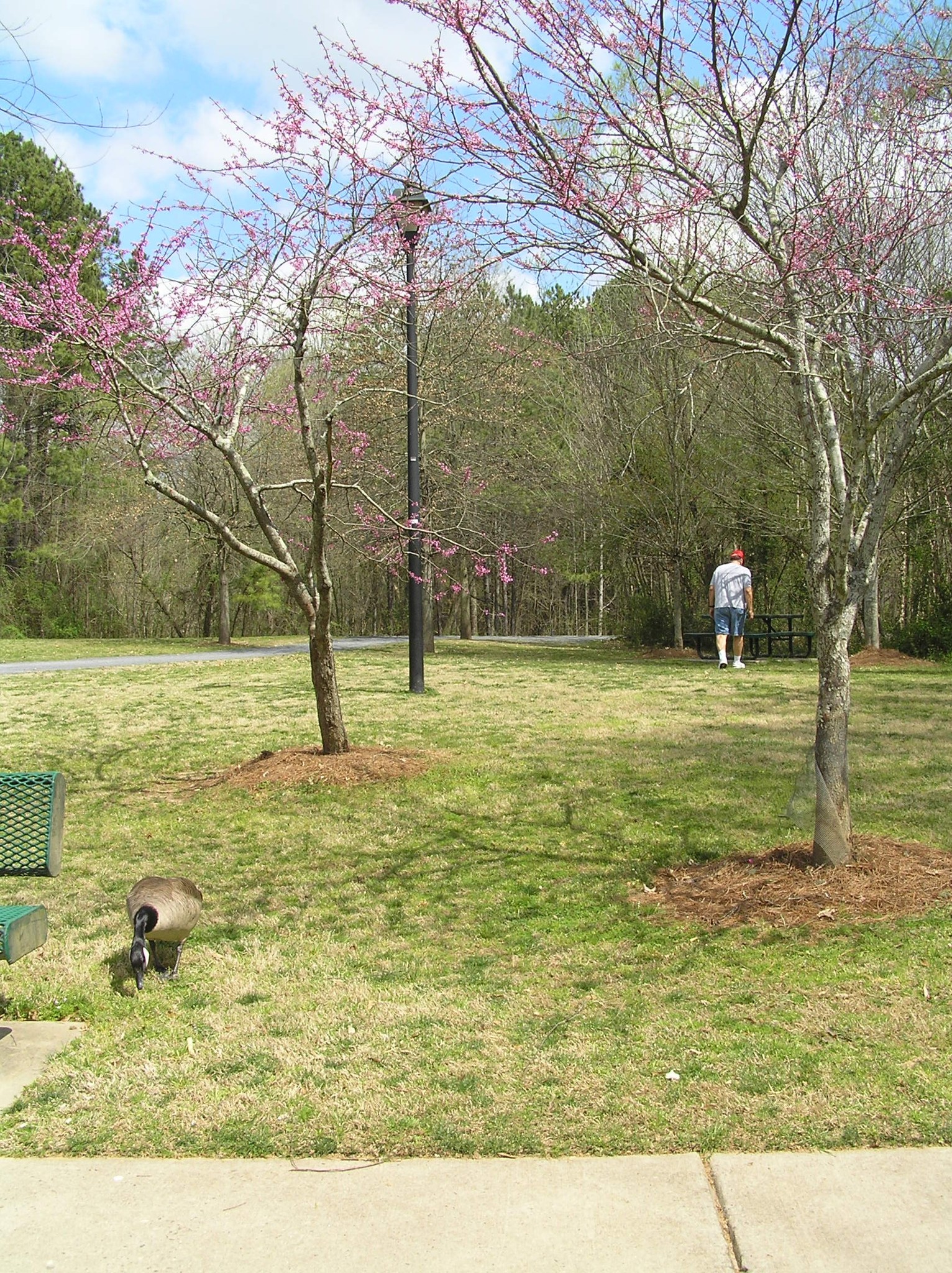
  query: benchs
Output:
[684,632,816,660]
[0,773,64,965]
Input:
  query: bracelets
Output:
[708,605,714,608]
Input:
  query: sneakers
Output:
[717,660,728,670]
[732,662,746,669]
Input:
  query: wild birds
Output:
[125,876,203,990]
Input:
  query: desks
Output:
[699,614,804,658]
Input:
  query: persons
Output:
[708,550,755,668]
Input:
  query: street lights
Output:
[386,182,436,694]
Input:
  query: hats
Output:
[732,549,745,559]
